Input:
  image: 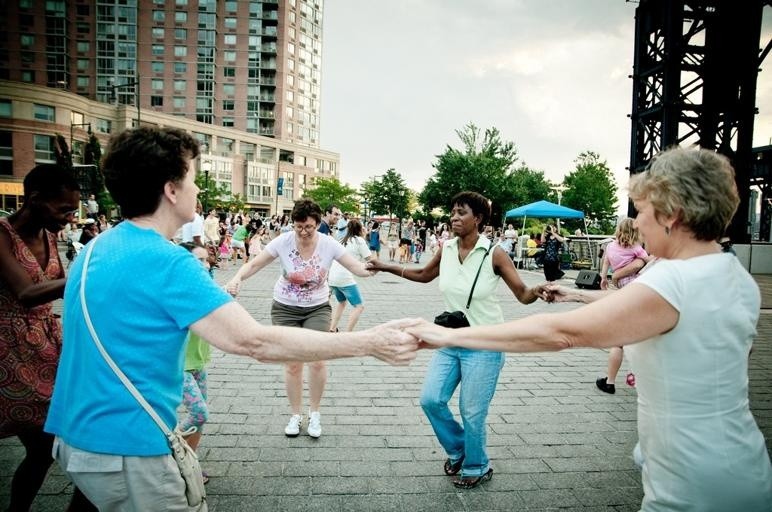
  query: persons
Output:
[505,223,519,250]
[82,194,100,221]
[540,225,566,282]
[260,228,273,250]
[439,223,452,246]
[404,147,772,512]
[399,238,406,263]
[536,233,542,246]
[241,211,251,225]
[600,217,650,290]
[57,212,74,242]
[415,237,424,263]
[275,215,281,229]
[270,214,279,231]
[278,214,293,235]
[365,221,373,246]
[226,222,240,242]
[336,212,349,241]
[217,222,227,237]
[220,236,230,271]
[595,258,646,395]
[236,216,242,226]
[79,217,97,244]
[0,163,99,512]
[41,127,421,512]
[231,224,253,265]
[226,199,380,439]
[247,228,264,261]
[429,231,437,255]
[249,211,262,237]
[173,241,238,484]
[496,233,515,260]
[317,205,341,235]
[182,198,205,247]
[369,222,385,259]
[417,217,428,251]
[204,208,220,247]
[364,190,555,489]
[526,234,544,269]
[97,214,108,232]
[387,222,399,262]
[486,229,494,242]
[328,220,373,332]
[406,240,411,263]
[402,221,415,262]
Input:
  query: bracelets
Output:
[401,267,406,278]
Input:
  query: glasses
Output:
[291,223,315,229]
[43,201,80,219]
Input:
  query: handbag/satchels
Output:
[80,236,206,506]
[433,240,494,329]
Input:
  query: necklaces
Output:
[459,240,476,262]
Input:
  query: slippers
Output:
[445,457,463,474]
[453,468,494,489]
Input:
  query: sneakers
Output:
[285,413,302,436]
[307,407,322,438]
[596,377,615,394]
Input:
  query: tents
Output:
[501,199,594,270]
